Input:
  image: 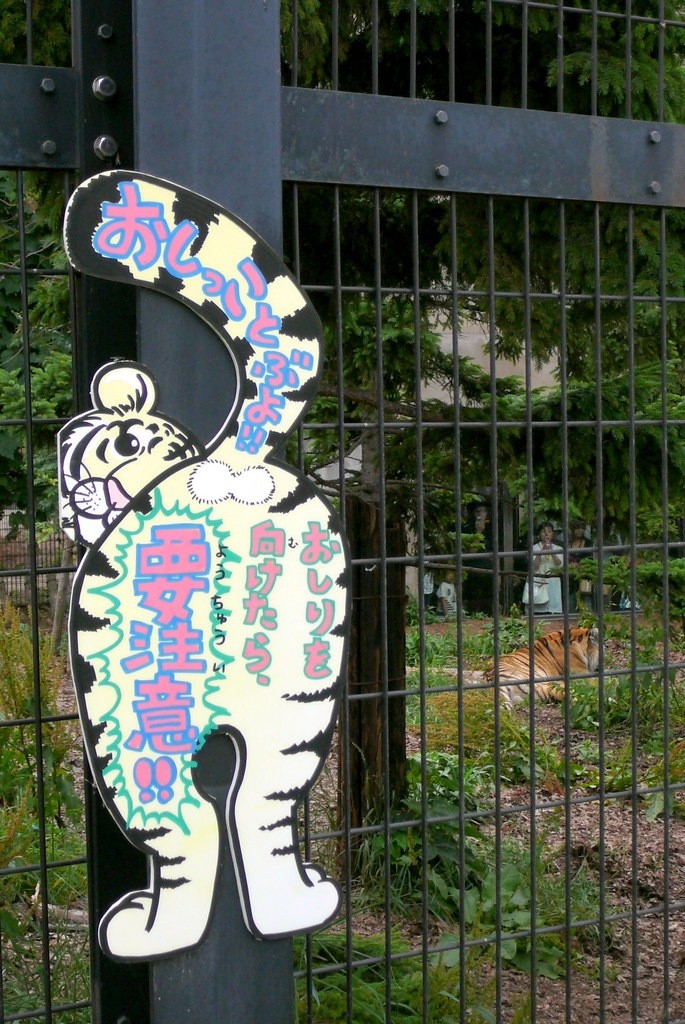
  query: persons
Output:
[525,522,564,614]
[423,500,622,615]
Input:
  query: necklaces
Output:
[538,542,551,560]
[570,539,582,548]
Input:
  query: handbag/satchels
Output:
[522,573,551,607]
